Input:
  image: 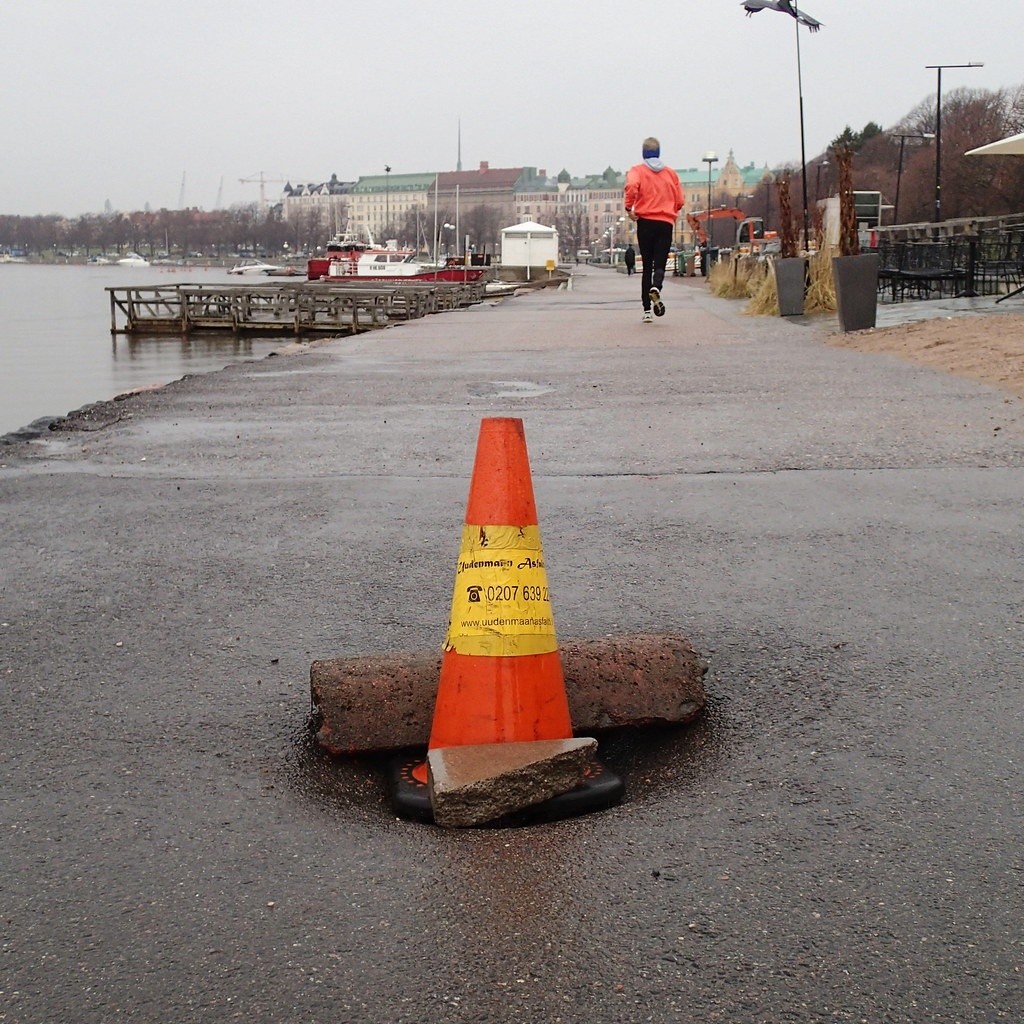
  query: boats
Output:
[307,201,487,285]
[116,254,150,268]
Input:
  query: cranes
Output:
[239,171,321,211]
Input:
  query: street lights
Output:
[815,159,830,202]
[925,60,985,264]
[616,217,625,265]
[384,164,391,237]
[444,223,456,265]
[591,239,599,258]
[702,150,720,273]
[762,182,786,230]
[731,193,754,245]
[892,133,936,230]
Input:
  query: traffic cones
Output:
[384,417,626,826]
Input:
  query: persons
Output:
[560,256,588,267]
[625,243,635,276]
[625,136,685,323]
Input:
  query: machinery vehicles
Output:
[687,204,777,255]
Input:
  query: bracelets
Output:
[625,206,632,212]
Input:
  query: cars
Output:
[176,249,275,259]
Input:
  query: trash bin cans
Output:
[700,246,731,276]
[686,255,695,276]
[770,256,808,317]
[831,251,880,332]
[678,254,686,273]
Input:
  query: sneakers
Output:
[649,287,665,316]
[641,310,653,322]
